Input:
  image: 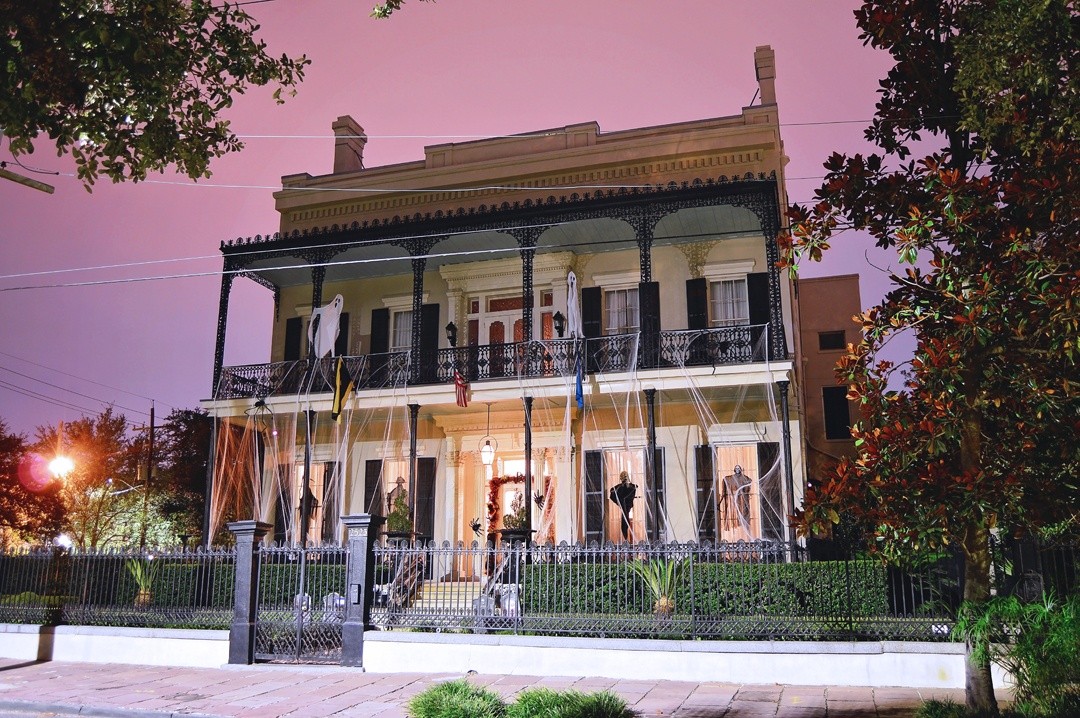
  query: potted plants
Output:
[495,506,538,565]
[376,488,423,566]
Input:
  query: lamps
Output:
[445,321,457,347]
[478,402,498,465]
[552,311,566,338]
